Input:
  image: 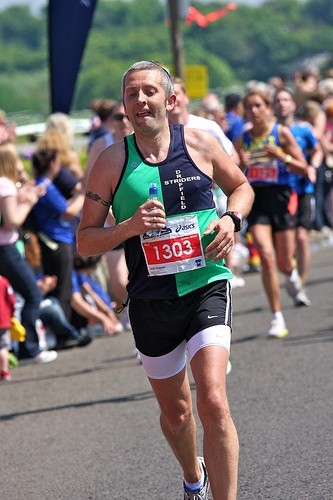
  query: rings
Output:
[153,217,156,223]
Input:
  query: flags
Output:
[48,0,99,113]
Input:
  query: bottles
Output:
[87,324,125,338]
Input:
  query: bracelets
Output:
[284,154,291,164]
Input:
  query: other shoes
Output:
[19,350,57,364]
[89,323,114,337]
[267,318,288,338]
[285,270,302,297]
[59,336,92,349]
[294,292,310,307]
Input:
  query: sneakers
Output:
[182,456,210,500]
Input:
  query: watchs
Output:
[220,211,244,232]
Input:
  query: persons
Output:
[167,78,239,375]
[234,93,308,339]
[0,98,142,381]
[191,68,333,289]
[272,89,324,307]
[76,61,255,500]
